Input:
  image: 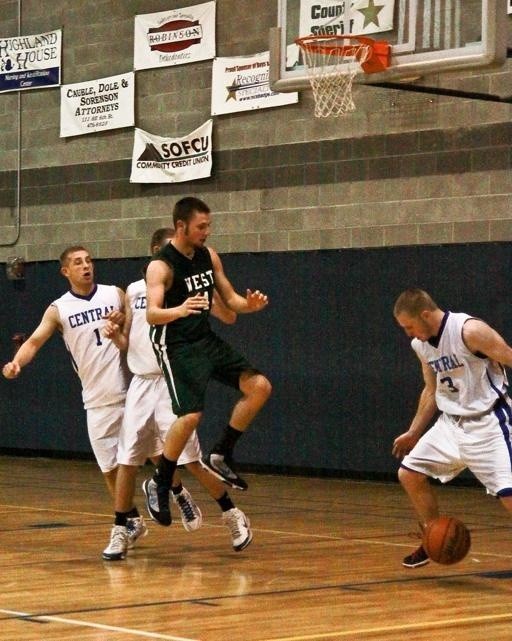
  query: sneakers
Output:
[173,486,204,534]
[402,545,432,569]
[142,478,173,527]
[199,450,250,492]
[222,507,254,553]
[103,524,130,561]
[126,515,150,551]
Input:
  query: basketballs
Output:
[421,515,476,564]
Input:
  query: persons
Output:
[4,246,202,551]
[142,196,272,528]
[103,228,256,559]
[392,289,512,568]
